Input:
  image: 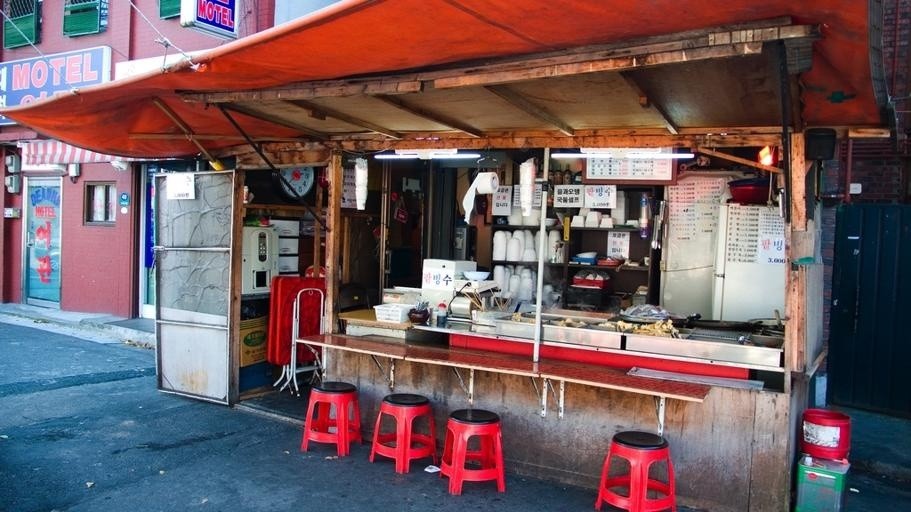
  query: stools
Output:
[369,393,438,474]
[439,409,507,496]
[594,431,677,511]
[300,381,363,457]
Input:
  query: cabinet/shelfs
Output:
[489,183,662,315]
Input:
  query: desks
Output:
[295,334,709,437]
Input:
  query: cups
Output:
[492,229,561,295]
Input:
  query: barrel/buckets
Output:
[801,406,851,461]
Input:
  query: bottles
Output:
[548,163,573,185]
[407,309,430,323]
[436,302,447,327]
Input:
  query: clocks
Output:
[279,167,314,199]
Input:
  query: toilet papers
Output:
[463,172,499,224]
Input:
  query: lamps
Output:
[549,152,697,159]
[757,145,779,168]
[372,153,481,161]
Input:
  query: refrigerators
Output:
[712,205,824,367]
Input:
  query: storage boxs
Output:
[239,315,268,368]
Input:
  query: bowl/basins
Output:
[538,218,558,227]
[462,271,491,280]
[727,176,776,206]
[576,251,597,259]
